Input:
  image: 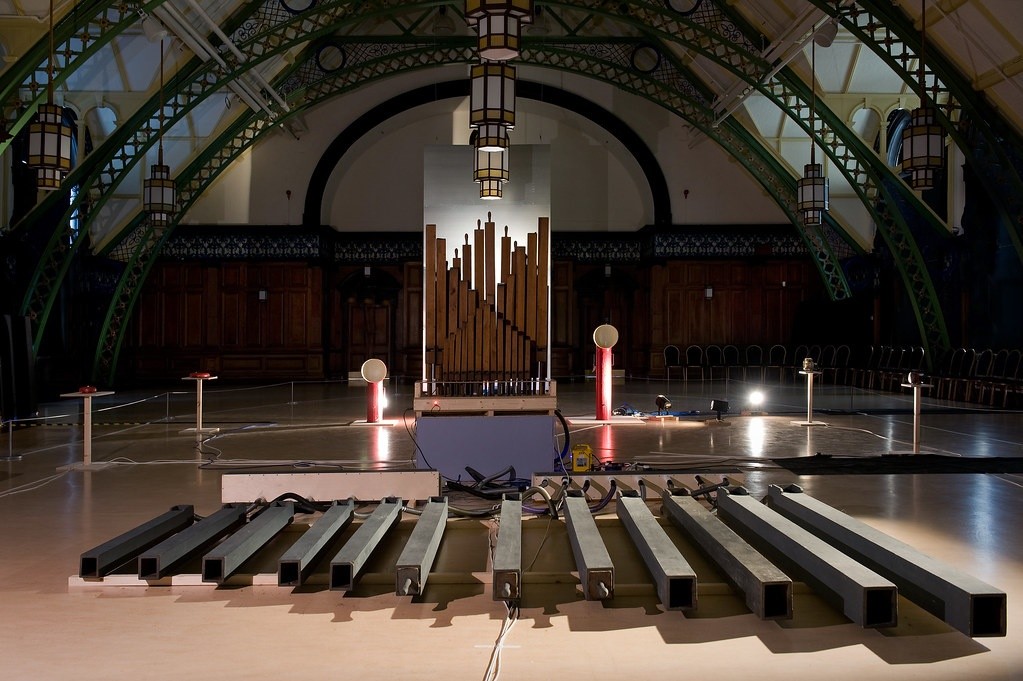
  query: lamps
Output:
[27,0,71,191]
[473,131,511,200]
[710,400,729,421]
[814,14,843,47]
[798,24,829,226]
[469,54,517,153]
[464,0,535,61]
[143,21,177,228]
[903,0,945,191]
[656,395,674,418]
[137,8,167,43]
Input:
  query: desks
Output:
[901,383,934,454]
[59,392,115,472]
[795,371,830,426]
[178,376,220,433]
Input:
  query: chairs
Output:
[705,344,727,382]
[685,345,705,383]
[782,344,1023,407]
[723,345,745,382]
[664,345,685,382]
[743,344,766,382]
[766,344,787,385]
